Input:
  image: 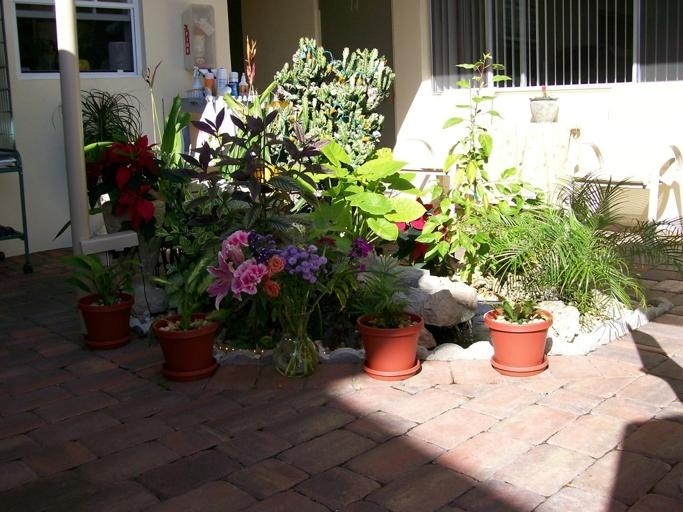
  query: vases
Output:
[530,100,558,123]
[273,312,320,379]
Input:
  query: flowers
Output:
[206,230,374,376]
[542,84,548,98]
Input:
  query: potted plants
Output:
[482,295,554,377]
[345,255,425,381]
[146,258,233,381]
[61,254,143,350]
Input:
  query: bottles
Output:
[203,66,249,96]
